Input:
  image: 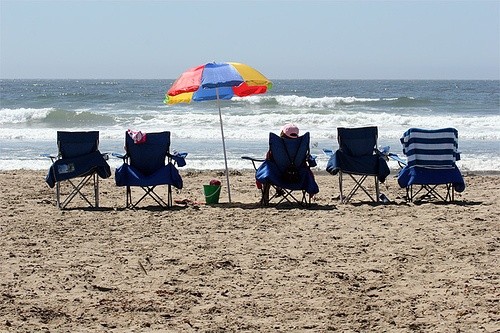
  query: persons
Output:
[280,123,300,138]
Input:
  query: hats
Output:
[282,124,300,138]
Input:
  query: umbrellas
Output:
[163,61,274,202]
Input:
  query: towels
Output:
[209,179,221,186]
[127,127,148,145]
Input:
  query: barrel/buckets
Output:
[203,184,221,204]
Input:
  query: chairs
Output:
[240,131,320,209]
[108,130,187,210]
[44,129,111,209]
[325,125,391,206]
[388,126,466,205]
